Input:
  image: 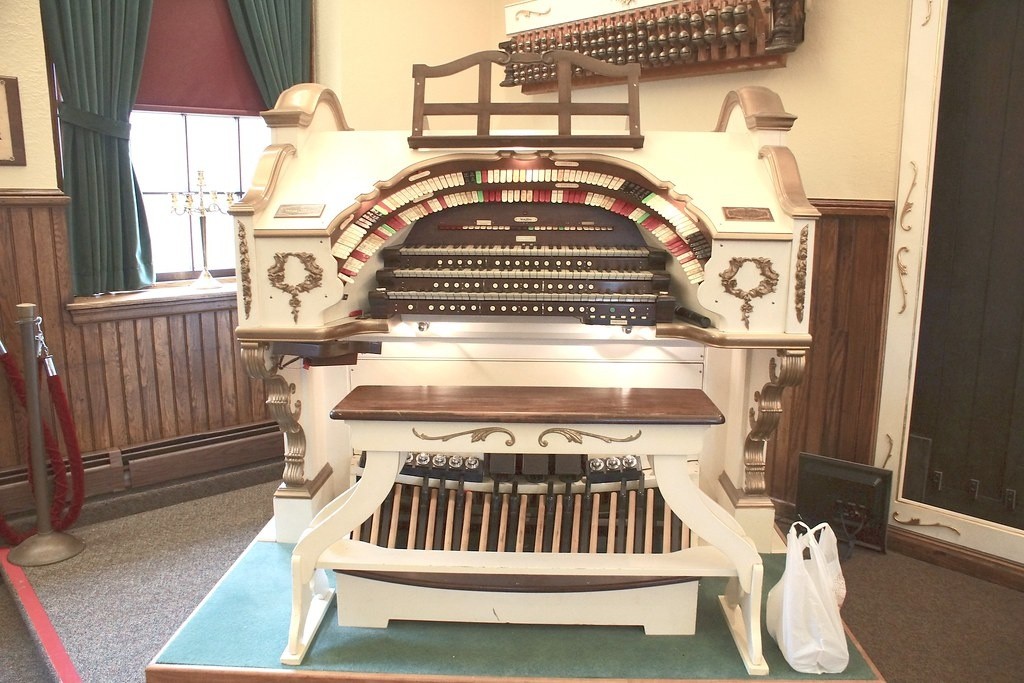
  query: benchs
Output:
[274,381,782,677]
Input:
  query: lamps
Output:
[168,169,238,290]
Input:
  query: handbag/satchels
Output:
[765,520,850,674]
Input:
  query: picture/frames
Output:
[0,75,28,167]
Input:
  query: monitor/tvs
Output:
[794,452,895,549]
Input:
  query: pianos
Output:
[223,37,824,679]
